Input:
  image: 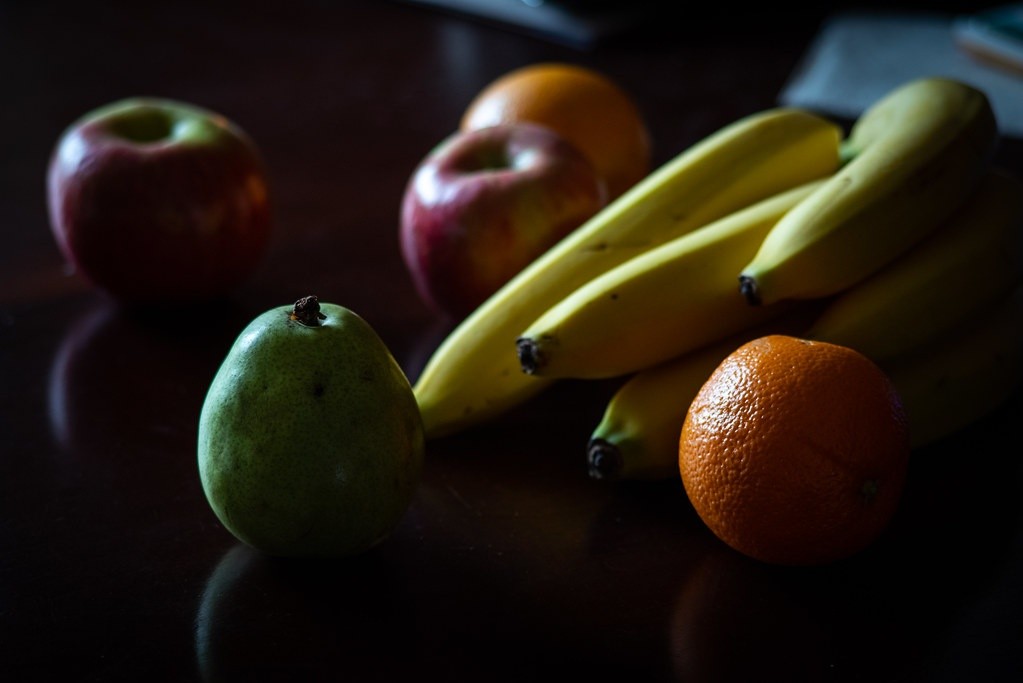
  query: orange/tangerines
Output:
[678,333,910,567]
[460,58,653,204]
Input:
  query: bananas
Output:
[411,76,1023,484]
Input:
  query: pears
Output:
[196,294,427,564]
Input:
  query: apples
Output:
[393,125,611,326]
[46,95,273,313]
